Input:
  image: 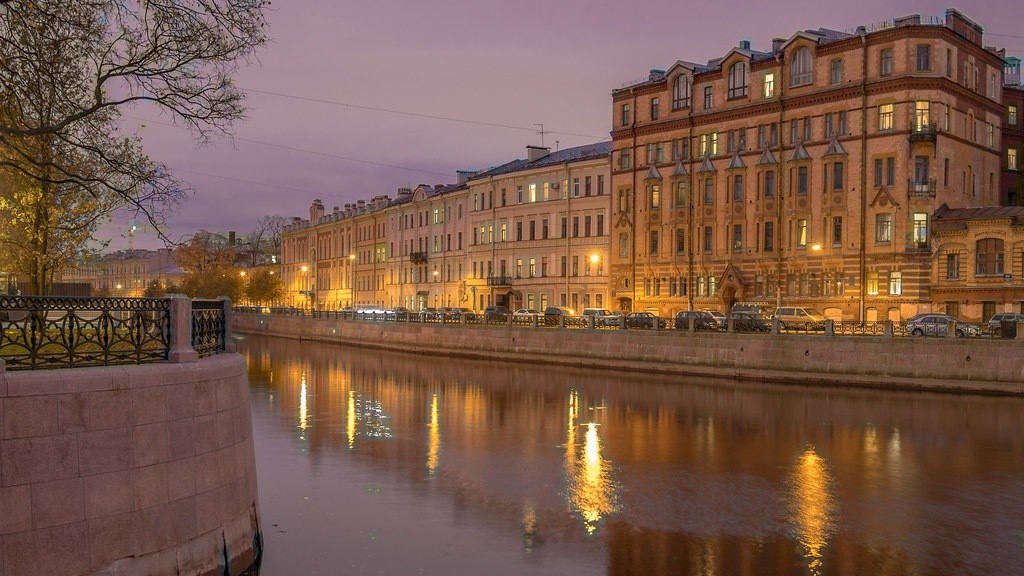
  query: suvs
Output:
[987,312,1024,336]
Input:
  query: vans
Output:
[774,306,829,331]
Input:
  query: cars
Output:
[907,315,982,338]
[234,299,774,332]
